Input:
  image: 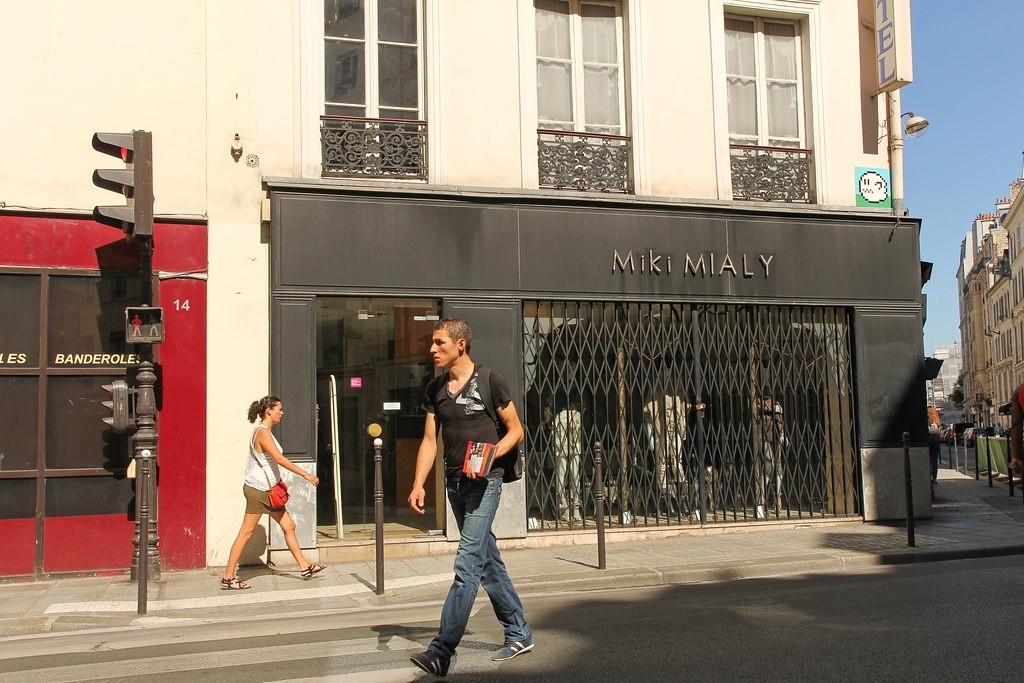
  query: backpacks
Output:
[435,367,522,483]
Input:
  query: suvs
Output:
[960,427,986,448]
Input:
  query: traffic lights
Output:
[92,129,153,242]
[99,380,128,435]
[126,304,165,344]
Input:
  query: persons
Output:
[929,420,942,484]
[756,384,790,513]
[1008,382,1024,475]
[220,395,327,590]
[406,317,534,679]
[551,400,585,522]
[642,380,693,516]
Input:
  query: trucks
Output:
[941,422,974,445]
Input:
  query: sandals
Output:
[301,564,326,580]
[221,578,251,590]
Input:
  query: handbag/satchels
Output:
[268,481,291,510]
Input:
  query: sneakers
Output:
[410,650,451,678]
[491,628,534,661]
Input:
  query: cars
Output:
[992,426,1004,436]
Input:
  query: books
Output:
[462,441,499,477]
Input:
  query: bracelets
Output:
[303,472,306,477]
